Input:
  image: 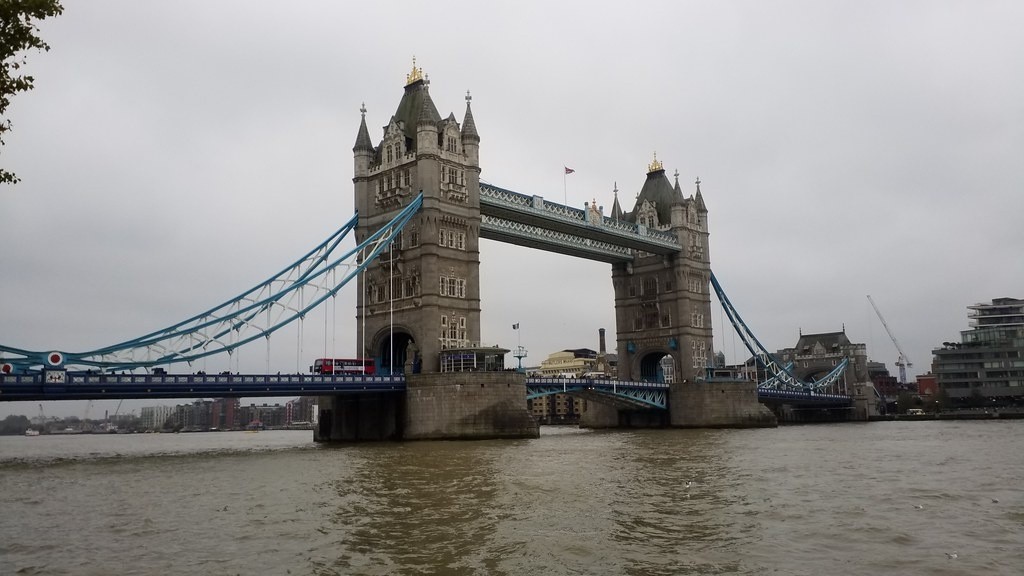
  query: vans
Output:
[908,409,925,415]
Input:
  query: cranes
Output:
[866,294,913,382]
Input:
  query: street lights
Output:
[993,399,996,410]
[936,401,938,412]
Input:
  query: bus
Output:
[314,358,376,375]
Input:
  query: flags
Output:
[565,167,575,175]
[513,323,519,330]
